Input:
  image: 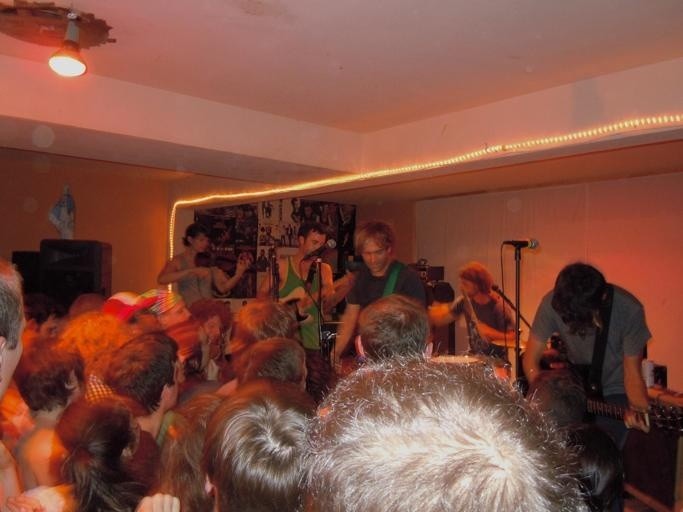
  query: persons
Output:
[328,220,427,374]
[155,222,254,315]
[196,201,355,303]
[525,263,653,483]
[1,256,624,512]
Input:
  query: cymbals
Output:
[492,339,527,349]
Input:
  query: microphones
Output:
[504,239,539,248]
[304,240,336,260]
[492,285,515,312]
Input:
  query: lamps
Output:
[49,9,88,79]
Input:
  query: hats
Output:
[102,291,157,321]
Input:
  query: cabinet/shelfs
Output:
[11,249,40,322]
[39,238,112,321]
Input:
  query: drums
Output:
[433,354,478,365]
[485,358,512,382]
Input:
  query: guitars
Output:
[520,348,683,436]
[275,269,359,326]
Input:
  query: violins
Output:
[195,239,268,272]
